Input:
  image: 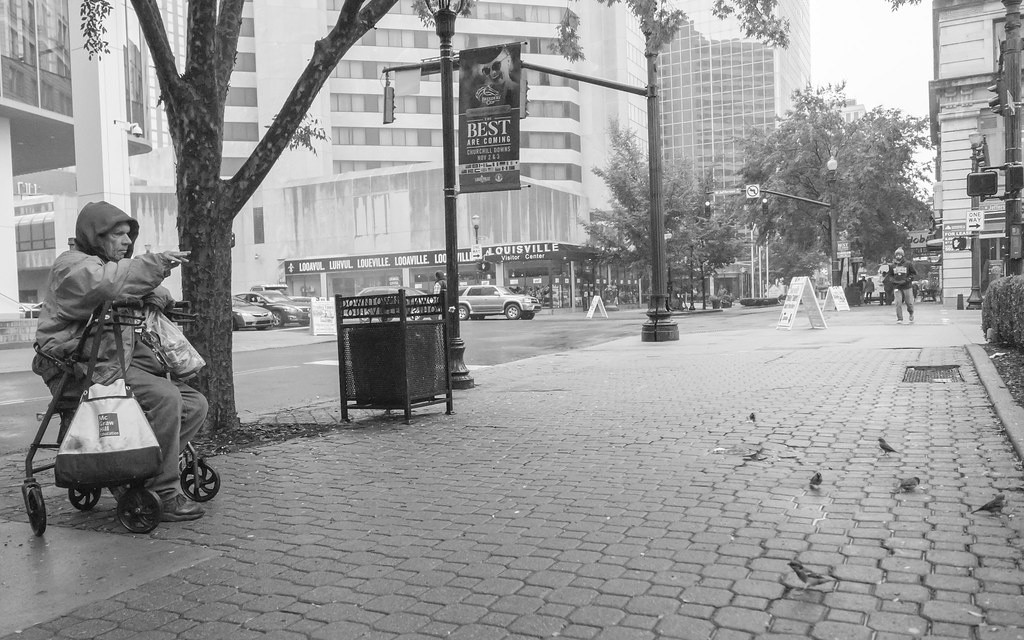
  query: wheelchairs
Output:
[21,295,220,537]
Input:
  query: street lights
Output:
[965,129,985,309]
[826,155,839,287]
[425,0,475,391]
[471,214,480,245]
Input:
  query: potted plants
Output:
[710,296,721,309]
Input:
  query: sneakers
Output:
[160,493,206,522]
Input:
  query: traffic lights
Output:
[952,237,961,249]
[761,197,768,216]
[704,200,711,219]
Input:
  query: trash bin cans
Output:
[331,288,455,423]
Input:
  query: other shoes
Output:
[909,314,914,321]
[897,319,902,324]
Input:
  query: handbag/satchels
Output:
[53,378,164,488]
[139,306,207,382]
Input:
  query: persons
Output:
[877,271,893,306]
[718,285,728,296]
[31,200,206,522]
[433,270,447,294]
[861,276,874,304]
[893,247,916,323]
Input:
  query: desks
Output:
[920,290,936,302]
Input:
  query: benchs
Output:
[866,292,885,304]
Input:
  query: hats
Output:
[895,247,904,257]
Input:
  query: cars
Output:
[858,276,929,302]
[231,283,318,330]
[20,302,44,319]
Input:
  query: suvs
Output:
[457,286,542,320]
[357,285,440,321]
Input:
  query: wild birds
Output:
[786,561,842,592]
[877,437,899,456]
[889,477,920,495]
[809,472,822,486]
[749,413,755,422]
[970,494,1007,515]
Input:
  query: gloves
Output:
[898,280,907,286]
[890,277,895,284]
